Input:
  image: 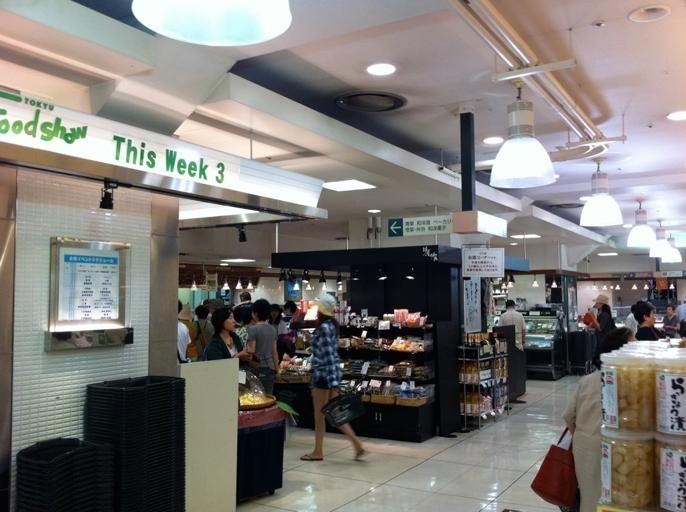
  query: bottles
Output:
[458,331,508,415]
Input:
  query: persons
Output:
[498,299,526,350]
[299,294,369,462]
[177,290,300,394]
[586,295,686,370]
[563,327,638,512]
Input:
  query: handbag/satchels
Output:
[320,388,368,427]
[531,427,578,506]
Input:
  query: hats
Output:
[592,295,608,304]
[313,293,337,317]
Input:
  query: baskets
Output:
[371,394,434,407]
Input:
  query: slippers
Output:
[300,454,322,460]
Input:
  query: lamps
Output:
[488,78,559,190]
[551,276,558,289]
[275,267,418,286]
[94,179,117,212]
[579,156,623,229]
[649,217,674,258]
[626,199,657,249]
[660,233,682,264]
[590,280,675,291]
[236,226,249,245]
[499,272,515,290]
[531,275,539,288]
[131,0,295,48]
